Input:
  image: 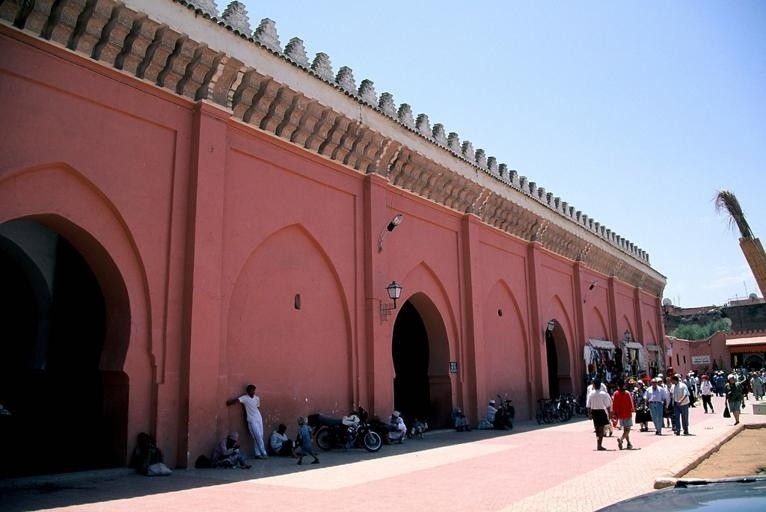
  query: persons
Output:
[452,408,470,431]
[294,415,321,465]
[385,411,407,444]
[130,432,165,469]
[211,432,252,470]
[226,384,269,459]
[488,399,500,425]
[409,417,428,439]
[579,357,765,452]
[268,423,297,457]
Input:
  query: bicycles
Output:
[497,394,515,419]
[535,392,580,425]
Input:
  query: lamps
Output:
[622,329,631,344]
[379,280,403,321]
[376,214,402,255]
[542,320,559,341]
[582,280,597,303]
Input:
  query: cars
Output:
[595,477,766,511]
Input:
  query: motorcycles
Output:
[308,406,383,452]
